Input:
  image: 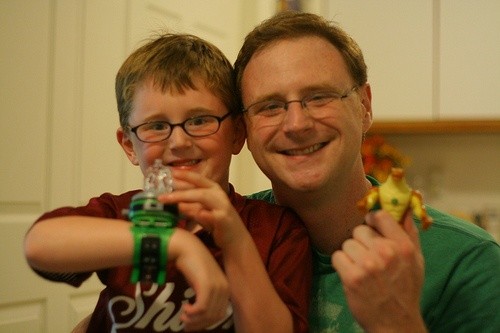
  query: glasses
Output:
[125,111,231,143]
[240,83,361,118]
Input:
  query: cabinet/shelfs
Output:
[295,0,499,134]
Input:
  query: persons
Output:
[231,9,500,333]
[21,29,316,332]
